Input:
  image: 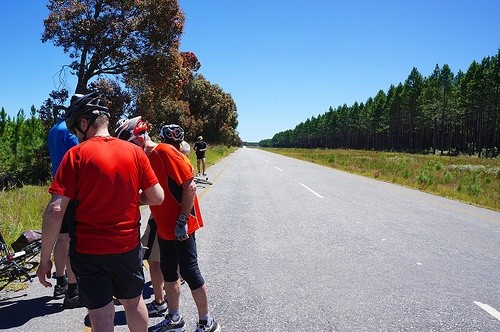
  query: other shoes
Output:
[203,172,207,175]
[62,289,83,307]
[196,173,200,176]
[54,284,68,298]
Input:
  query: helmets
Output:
[160,123,185,145]
[64,92,110,135]
[113,115,150,149]
[197,136,204,140]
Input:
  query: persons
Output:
[36,92,165,331]
[193,136,207,176]
[114,115,221,332]
[141,123,185,318]
[46,93,87,309]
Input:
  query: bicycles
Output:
[193,177,212,188]
[0,234,43,280]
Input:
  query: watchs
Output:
[178,214,188,223]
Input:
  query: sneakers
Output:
[146,300,169,316]
[146,313,186,332]
[192,318,221,332]
